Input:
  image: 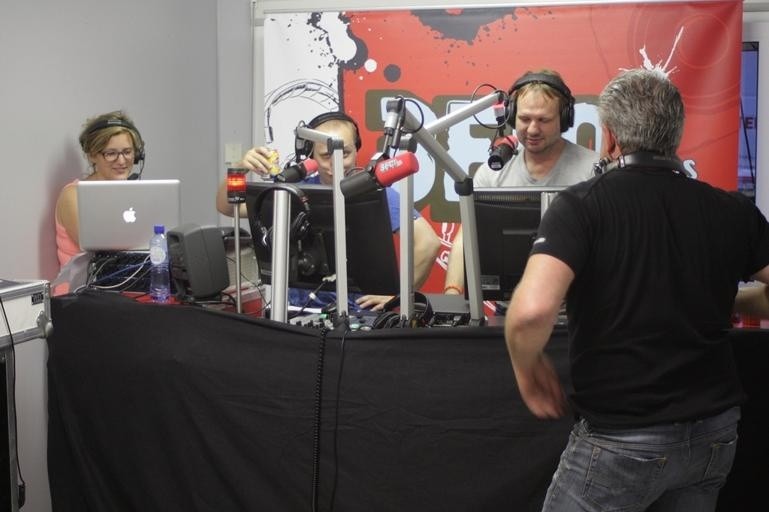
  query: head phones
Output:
[594,149,687,177]
[292,113,361,162]
[371,291,432,330]
[505,73,575,132]
[251,184,312,251]
[80,120,145,164]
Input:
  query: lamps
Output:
[225,168,250,316]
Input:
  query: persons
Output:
[503,65,768,512]
[441,68,608,297]
[216,108,442,314]
[52,106,182,306]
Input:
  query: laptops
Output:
[76,179,181,251]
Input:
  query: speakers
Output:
[167,223,230,304]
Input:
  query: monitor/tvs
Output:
[244,182,400,295]
[465,186,571,326]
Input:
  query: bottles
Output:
[149,225,171,300]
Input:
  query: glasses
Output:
[99,146,136,162]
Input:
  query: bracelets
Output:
[442,284,465,296]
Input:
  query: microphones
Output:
[284,155,297,169]
[297,240,305,267]
[340,152,419,199]
[488,135,518,171]
[127,154,144,179]
[275,159,318,181]
